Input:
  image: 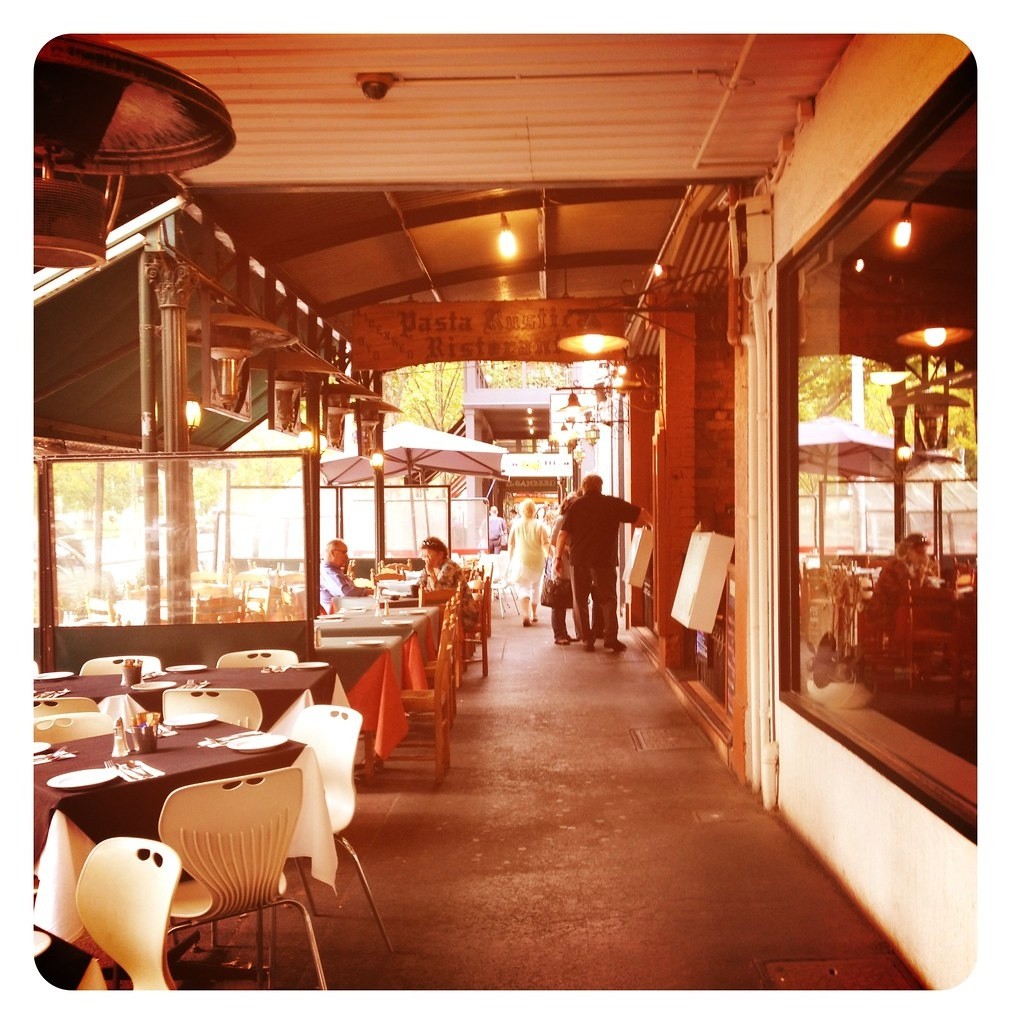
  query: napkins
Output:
[197,730,264,749]
[142,670,168,679]
[34,750,77,765]
[178,680,210,690]
[261,664,291,673]
[113,760,166,782]
[127,724,177,737]
[34,688,70,699]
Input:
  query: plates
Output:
[34,742,51,754]
[356,609,427,646]
[131,681,176,690]
[46,768,118,790]
[34,671,74,680]
[165,664,208,674]
[316,607,366,619]
[226,734,289,752]
[289,662,329,671]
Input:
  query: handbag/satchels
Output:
[540,576,573,609]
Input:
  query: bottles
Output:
[110,717,130,757]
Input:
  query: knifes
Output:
[104,760,136,782]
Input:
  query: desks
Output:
[314,635,410,778]
[321,615,430,693]
[34,719,339,990]
[34,664,353,738]
[370,595,419,608]
[348,605,440,658]
[114,599,208,626]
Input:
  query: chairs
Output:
[34,551,521,990]
[800,559,977,718]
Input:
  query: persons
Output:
[319,539,376,614]
[480,505,507,555]
[867,533,940,658]
[445,513,470,547]
[480,475,654,651]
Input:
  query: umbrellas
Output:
[800,418,909,487]
[318,421,507,557]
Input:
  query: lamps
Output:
[33,34,404,473]
[550,265,729,453]
[888,303,975,447]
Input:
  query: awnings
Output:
[402,416,465,487]
[447,474,468,499]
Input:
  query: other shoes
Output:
[533,617,538,622]
[585,646,594,651]
[604,640,627,650]
[523,617,533,626]
[555,634,579,645]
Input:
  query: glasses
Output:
[335,550,347,554]
[422,540,437,547]
